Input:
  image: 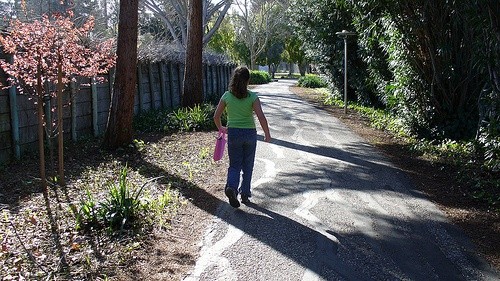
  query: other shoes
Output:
[241,193,249,203]
[226,187,240,208]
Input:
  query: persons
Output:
[213,66,272,209]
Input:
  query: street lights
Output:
[335,29,357,116]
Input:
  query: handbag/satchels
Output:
[214,127,227,161]
